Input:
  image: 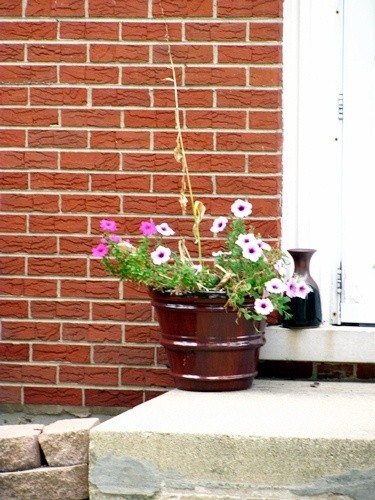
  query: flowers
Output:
[89,198,315,317]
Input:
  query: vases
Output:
[148,281,266,393]
[279,248,322,329]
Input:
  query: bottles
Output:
[282,248,322,327]
